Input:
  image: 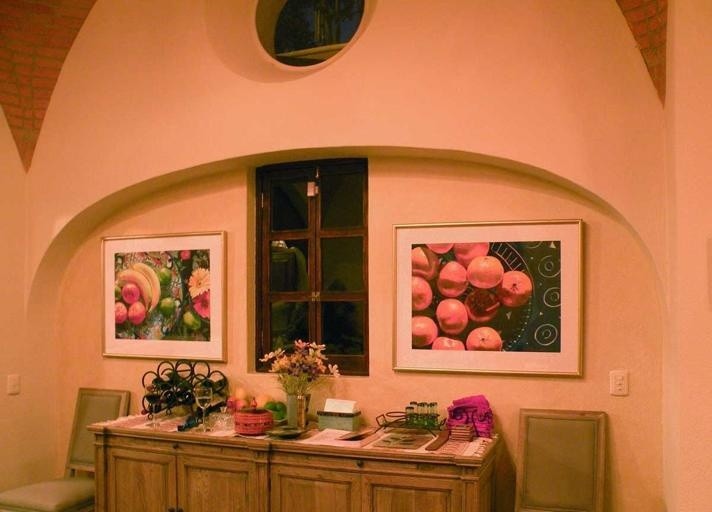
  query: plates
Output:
[264,428,306,438]
[273,415,288,425]
[412,242,534,351]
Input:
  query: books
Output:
[336,431,380,447]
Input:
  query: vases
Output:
[286,393,308,428]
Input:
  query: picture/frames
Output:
[390,217,587,378]
[99,229,228,363]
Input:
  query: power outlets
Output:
[610,369,629,396]
[7,374,22,395]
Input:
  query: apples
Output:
[183,311,201,331]
[160,296,176,316]
[160,268,172,286]
[265,401,287,412]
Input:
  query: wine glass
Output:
[194,387,213,433]
[145,384,161,428]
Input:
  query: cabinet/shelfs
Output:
[84,406,502,512]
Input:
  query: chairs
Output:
[515,408,606,512]
[0,387,131,512]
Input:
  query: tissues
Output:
[317,398,361,433]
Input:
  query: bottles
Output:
[405,401,439,429]
[251,396,257,409]
[215,405,236,434]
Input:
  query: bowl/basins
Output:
[235,409,274,435]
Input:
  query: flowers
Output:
[257,339,341,393]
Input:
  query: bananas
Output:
[120,263,160,319]
